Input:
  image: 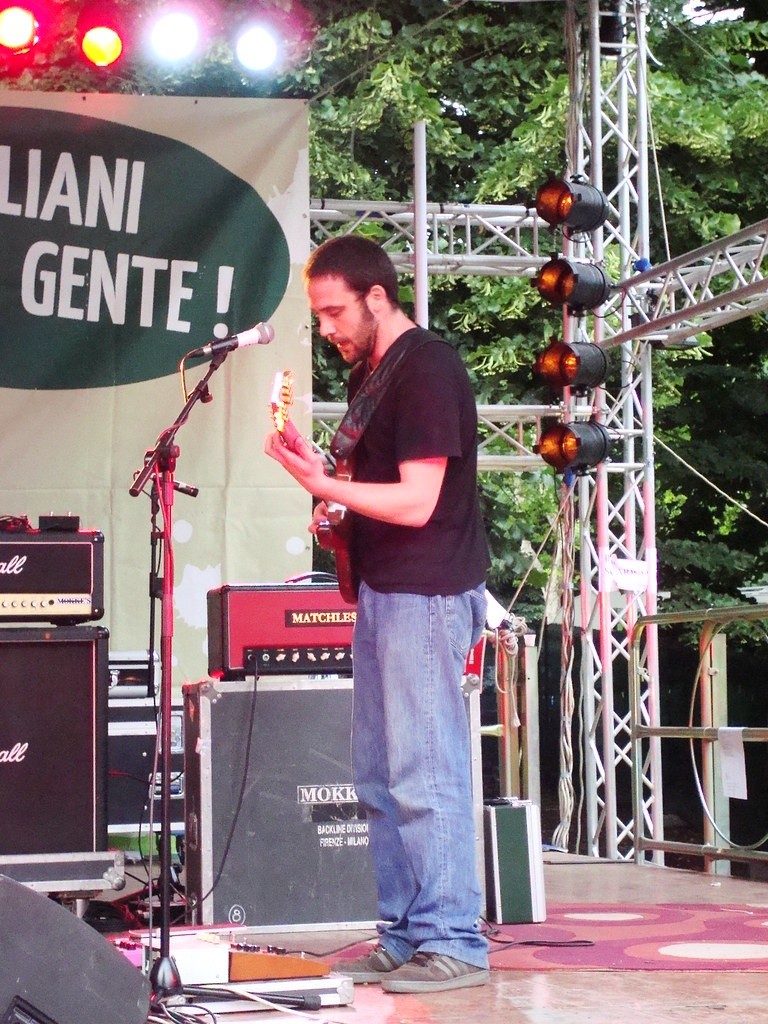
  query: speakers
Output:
[0,627,109,857]
[0,874,152,1024]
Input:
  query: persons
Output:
[264,236,490,994]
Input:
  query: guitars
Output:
[270,367,360,604]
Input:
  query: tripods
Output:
[130,353,322,1013]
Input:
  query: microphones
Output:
[189,322,275,359]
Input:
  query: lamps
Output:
[534,171,612,477]
[0,0,280,74]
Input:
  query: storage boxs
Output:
[482,797,547,924]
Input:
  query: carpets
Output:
[320,900,767,973]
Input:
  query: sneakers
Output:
[381,951,488,993]
[335,946,401,983]
